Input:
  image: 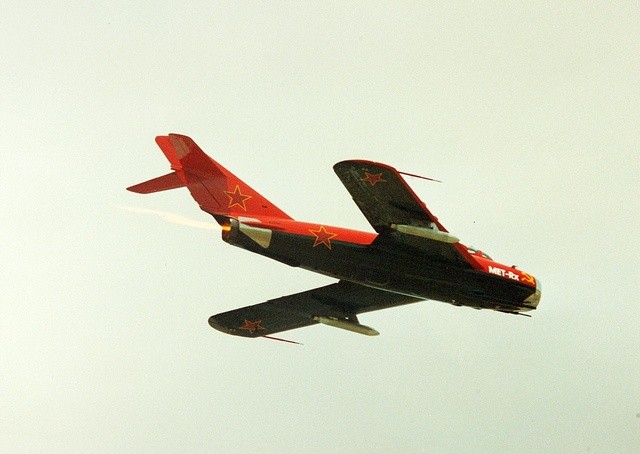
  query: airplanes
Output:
[127,133,542,346]
